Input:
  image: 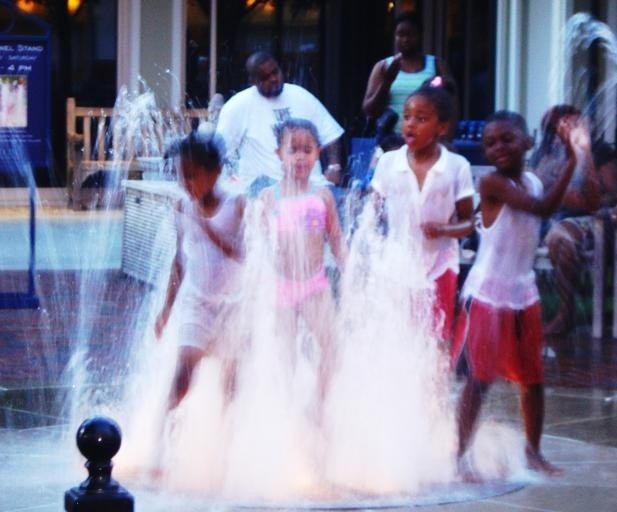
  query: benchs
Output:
[452,186,616,338]
[64,92,223,211]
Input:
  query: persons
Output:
[447,108,605,484]
[211,52,350,228]
[361,11,461,153]
[153,133,254,484]
[530,104,617,339]
[246,113,352,450]
[367,73,479,382]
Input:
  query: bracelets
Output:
[328,162,342,171]
